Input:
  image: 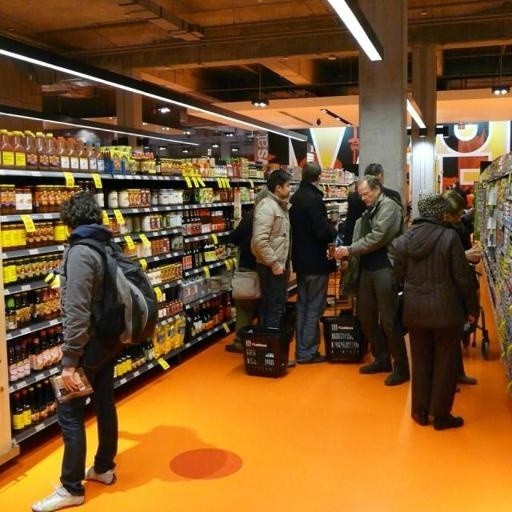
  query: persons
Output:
[223,160,411,386]
[443,187,486,387]
[391,190,481,432]
[30,190,127,512]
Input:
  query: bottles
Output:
[162,156,216,176]
[110,298,186,376]
[98,187,182,286]
[0,127,107,174]
[1,178,95,436]
[219,145,265,182]
[218,187,254,200]
[183,208,233,342]
[154,147,162,176]
[131,151,154,174]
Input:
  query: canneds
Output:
[1,221,72,252]
[0,184,81,216]
[3,252,66,288]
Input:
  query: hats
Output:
[417,192,450,216]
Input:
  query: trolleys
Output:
[460,266,490,359]
[326,266,353,315]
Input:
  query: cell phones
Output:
[328,243,335,257]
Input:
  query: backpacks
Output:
[64,239,156,351]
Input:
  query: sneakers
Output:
[411,411,464,429]
[84,465,114,484]
[384,374,410,385]
[224,344,241,352]
[455,376,477,391]
[282,352,325,367]
[31,484,84,512]
[359,364,393,373]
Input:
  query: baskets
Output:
[321,316,365,364]
[236,326,294,377]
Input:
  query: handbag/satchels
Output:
[231,270,264,301]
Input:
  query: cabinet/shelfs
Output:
[471,153,512,391]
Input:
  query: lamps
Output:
[407,92,427,130]
[324,1,384,63]
[250,65,269,106]
[156,105,172,115]
[492,85,510,96]
